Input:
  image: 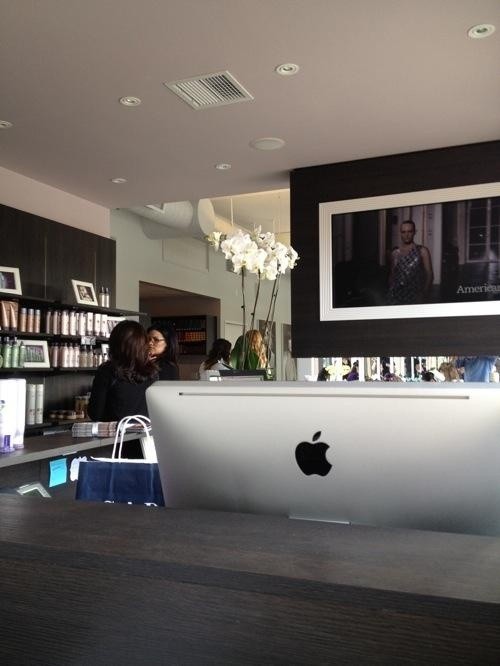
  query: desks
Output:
[0,420,153,496]
[0,496,500,663]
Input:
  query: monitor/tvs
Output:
[144,380,500,539]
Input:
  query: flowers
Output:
[205,224,303,372]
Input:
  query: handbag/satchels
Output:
[76,415,165,505]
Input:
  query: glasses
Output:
[148,337,165,343]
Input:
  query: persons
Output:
[455,355,497,382]
[386,219,435,304]
[87,320,179,422]
[230,330,271,374]
[79,286,95,301]
[199,338,237,381]
[343,359,499,382]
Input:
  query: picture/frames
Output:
[288,135,500,361]
[71,278,99,306]
[0,265,24,296]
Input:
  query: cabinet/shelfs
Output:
[151,312,217,356]
[0,292,154,439]
